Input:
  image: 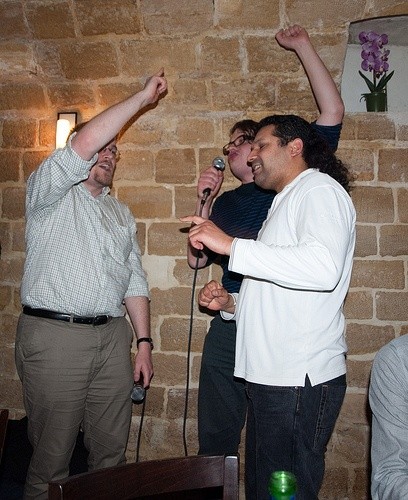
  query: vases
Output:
[366,92,387,112]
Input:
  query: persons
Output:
[187,25,346,500]
[179,114,358,500]
[15,67,166,500]
[368,333,408,500]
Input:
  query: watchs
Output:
[136,337,154,351]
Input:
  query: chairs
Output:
[48,451,239,500]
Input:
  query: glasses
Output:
[99,147,121,159]
[223,135,255,156]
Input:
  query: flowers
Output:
[358,31,395,102]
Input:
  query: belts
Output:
[22,305,112,328]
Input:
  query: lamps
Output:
[55,112,77,149]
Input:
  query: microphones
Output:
[130,370,146,402]
[202,156,225,201]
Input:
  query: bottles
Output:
[267,471,298,500]
